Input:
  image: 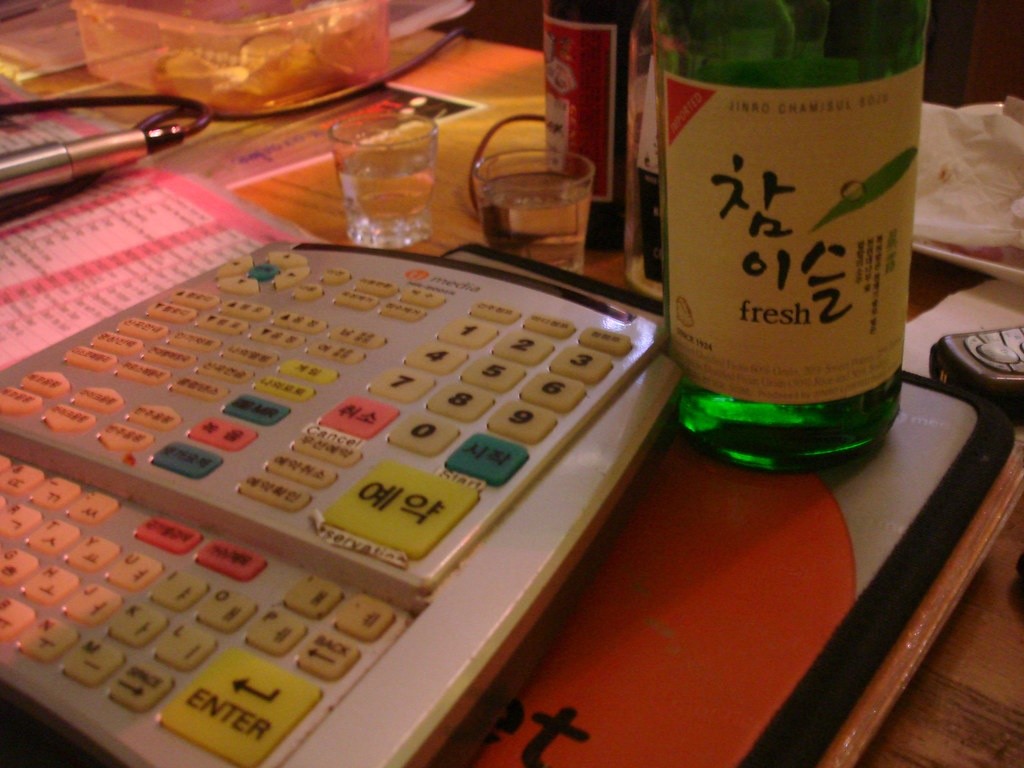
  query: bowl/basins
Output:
[66,0,396,115]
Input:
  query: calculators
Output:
[0,241,682,768]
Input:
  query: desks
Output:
[0,38,1024,767]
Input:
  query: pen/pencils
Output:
[0,122,184,198]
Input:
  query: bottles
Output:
[661,0,931,477]
[541,0,652,257]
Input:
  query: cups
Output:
[471,147,595,274]
[325,114,441,249]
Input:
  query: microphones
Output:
[0,124,183,226]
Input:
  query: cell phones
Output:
[929,326,1024,415]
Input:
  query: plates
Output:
[909,100,1023,289]
